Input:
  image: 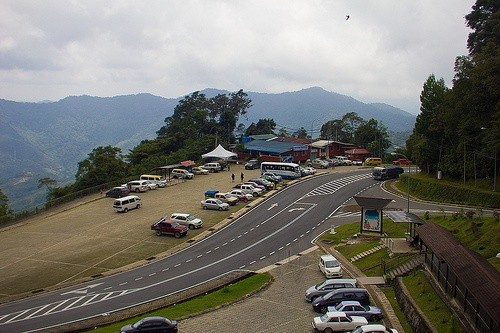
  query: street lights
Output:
[391,152,411,213]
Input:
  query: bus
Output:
[261,162,302,179]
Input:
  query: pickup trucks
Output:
[392,158,412,166]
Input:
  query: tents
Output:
[201,145,238,168]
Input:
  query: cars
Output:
[227,172,282,200]
[120,315,177,333]
[200,191,238,210]
[193,167,208,175]
[169,212,203,229]
[326,300,384,322]
[199,163,221,173]
[312,156,362,169]
[305,279,359,302]
[300,166,317,176]
[121,179,148,193]
[172,169,195,179]
[311,313,367,333]
[245,159,259,170]
[106,186,130,198]
[318,255,343,278]
[142,180,157,190]
[311,288,370,314]
[150,219,189,238]
[348,324,398,333]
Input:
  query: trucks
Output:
[372,166,405,180]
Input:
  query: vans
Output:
[140,175,167,187]
[113,195,143,214]
[365,158,383,166]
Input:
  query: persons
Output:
[231,173,235,182]
[228,163,230,171]
[241,172,244,181]
